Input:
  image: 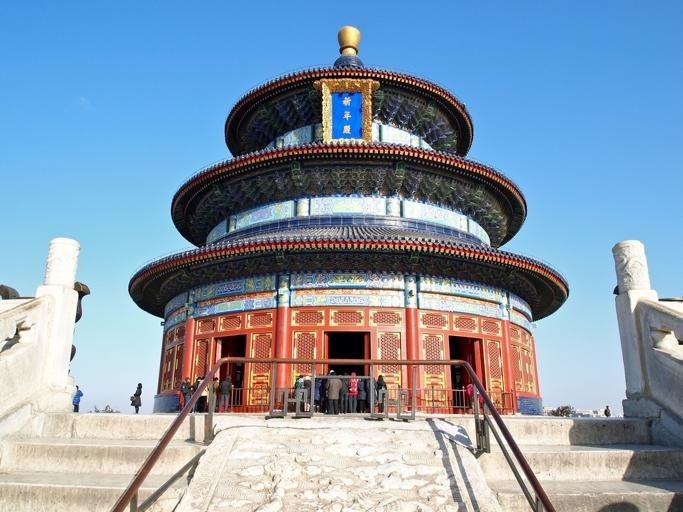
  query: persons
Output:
[183,375,232,413]
[466,384,483,414]
[604,405,611,417]
[131,382,142,414]
[294,369,387,413]
[72,385,83,412]
